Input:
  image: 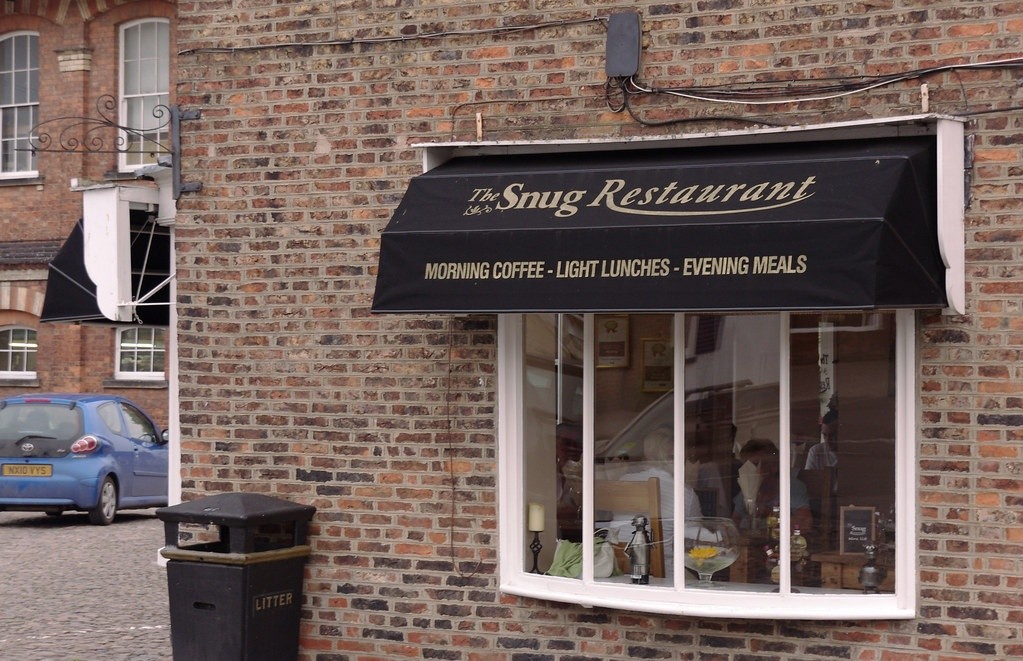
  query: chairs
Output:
[592,477,664,579]
[793,467,836,556]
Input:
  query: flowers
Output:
[689,545,720,566]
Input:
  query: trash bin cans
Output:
[153,491,317,661]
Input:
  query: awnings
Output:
[371,140,946,311]
[38,209,170,328]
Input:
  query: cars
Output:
[557,354,897,565]
[0,389,170,526]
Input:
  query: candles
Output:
[528,502,544,532]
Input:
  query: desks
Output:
[809,552,895,593]
[595,573,868,593]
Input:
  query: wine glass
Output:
[684,516,742,591]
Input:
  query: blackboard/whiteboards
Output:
[840,506,875,556]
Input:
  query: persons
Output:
[554,420,744,579]
[805,412,837,517]
[729,438,812,574]
